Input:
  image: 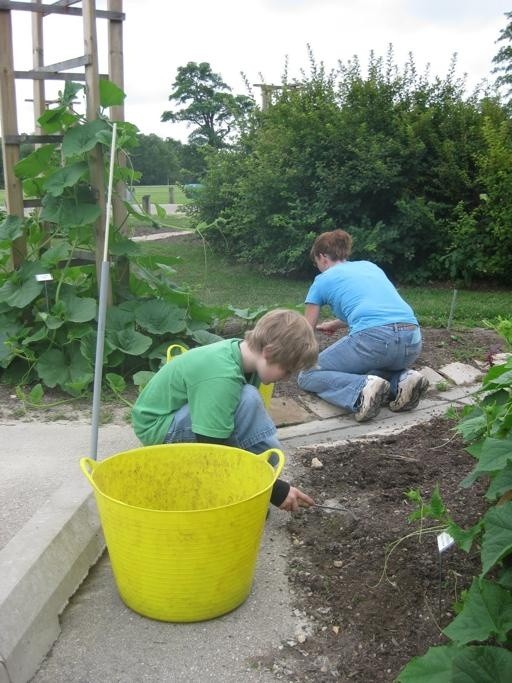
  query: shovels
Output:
[296,497,358,525]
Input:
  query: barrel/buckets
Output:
[81,441,285,623]
[165,343,276,413]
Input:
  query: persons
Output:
[131,309,319,513]
[297,229,430,422]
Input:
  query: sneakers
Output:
[389,369,429,413]
[354,375,390,422]
